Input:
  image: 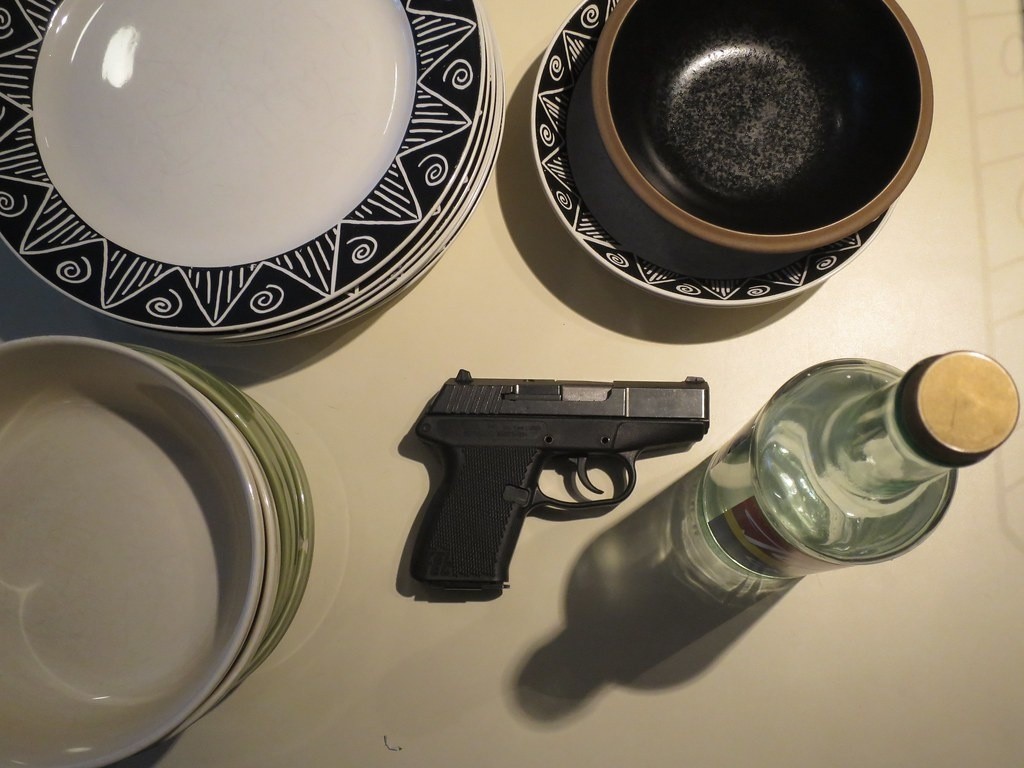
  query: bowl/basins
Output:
[595,0,936,254]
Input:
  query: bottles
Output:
[696,350,1020,582]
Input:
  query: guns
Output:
[408,368,714,596]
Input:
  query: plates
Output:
[0,0,506,349]
[531,0,895,308]
[0,332,317,768]
